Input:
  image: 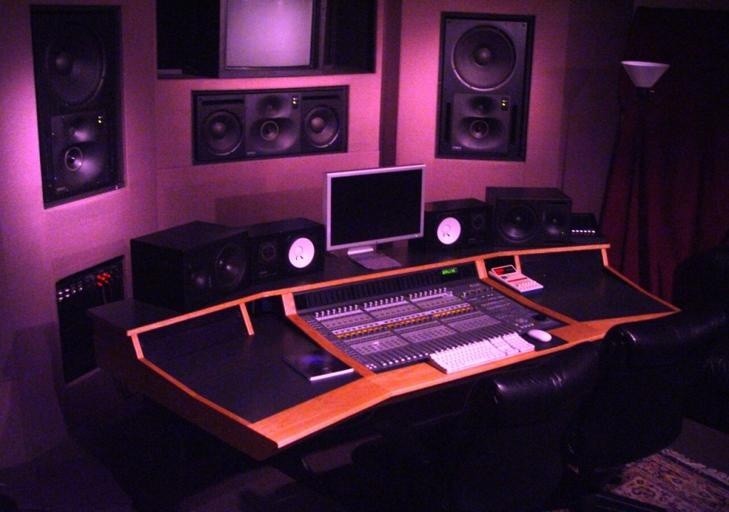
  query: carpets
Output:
[550,446,729,512]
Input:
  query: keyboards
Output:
[427,331,535,374]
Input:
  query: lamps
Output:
[619,60,669,294]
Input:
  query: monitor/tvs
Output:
[323,164,426,271]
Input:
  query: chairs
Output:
[585,310,729,512]
[369,343,606,512]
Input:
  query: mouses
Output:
[527,329,552,342]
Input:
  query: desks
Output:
[88,235,682,463]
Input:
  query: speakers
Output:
[239,217,325,287]
[130,220,249,313]
[192,0,328,78]
[30,4,125,209]
[438,14,535,160]
[486,186,572,246]
[191,85,348,166]
[408,198,488,254]
